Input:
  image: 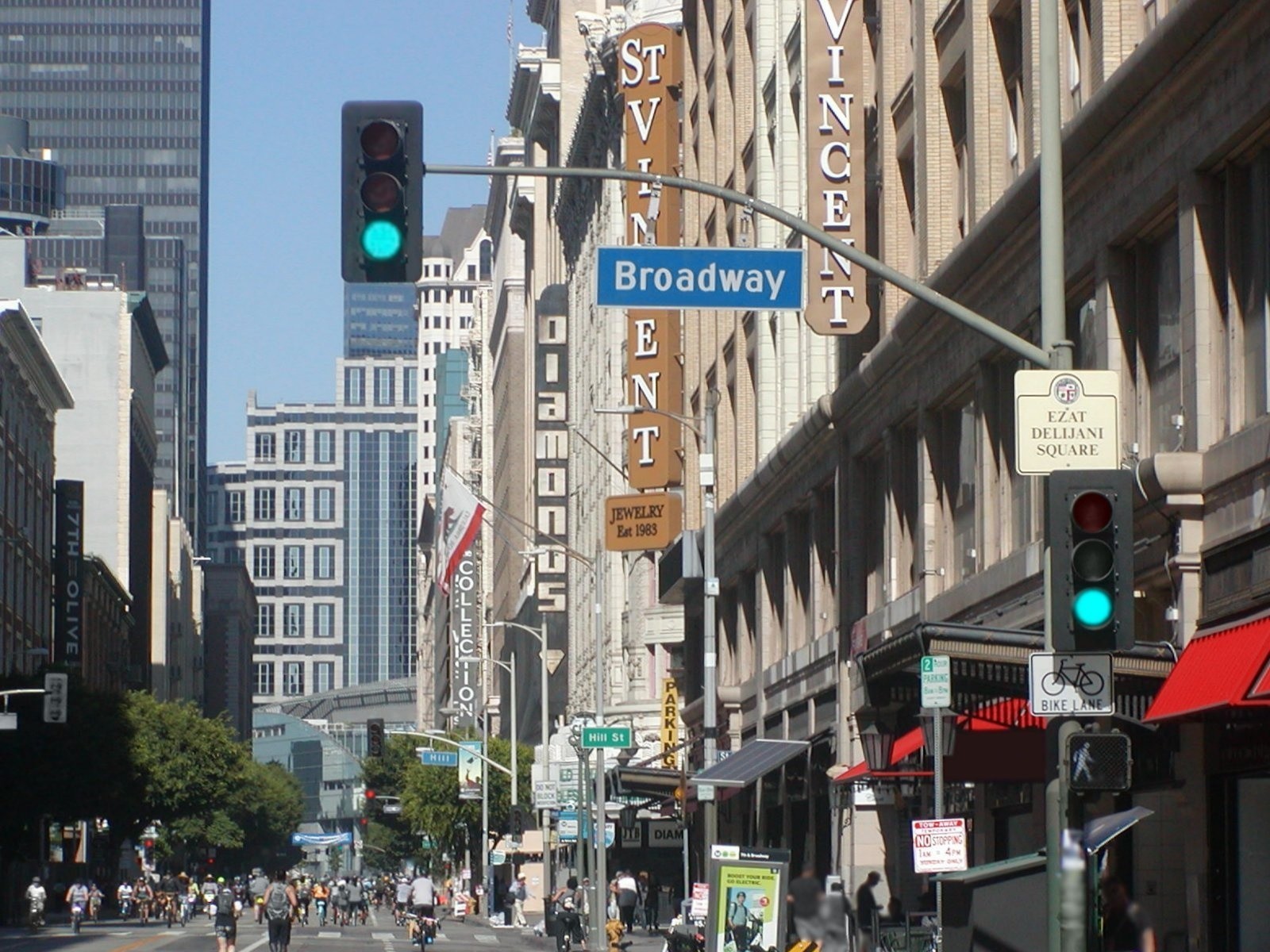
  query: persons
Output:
[583,877,589,925]
[552,876,588,952]
[261,869,298,952]
[25,876,47,927]
[115,868,434,928]
[728,892,753,952]
[1102,869,1156,952]
[607,867,659,933]
[213,888,236,952]
[788,861,883,952]
[446,876,453,898]
[410,868,441,946]
[668,863,691,918]
[66,878,105,922]
[509,871,536,928]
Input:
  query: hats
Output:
[32,876,40,882]
[736,892,746,898]
[136,870,242,884]
[518,873,525,881]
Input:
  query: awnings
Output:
[1140,608,1270,725]
[833,693,1047,784]
[494,830,569,855]
[592,740,810,818]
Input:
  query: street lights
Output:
[487,611,550,900]
[436,704,490,894]
[457,654,519,837]
[519,543,607,952]
[594,405,719,883]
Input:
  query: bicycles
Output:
[87,895,104,923]
[117,890,437,952]
[66,898,87,934]
[27,896,45,931]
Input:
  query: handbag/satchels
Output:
[506,892,517,905]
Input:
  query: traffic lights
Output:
[359,817,368,834]
[1049,467,1136,653]
[365,782,375,802]
[143,836,155,865]
[367,718,384,756]
[340,100,423,283]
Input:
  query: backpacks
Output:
[265,882,293,924]
[563,896,574,909]
